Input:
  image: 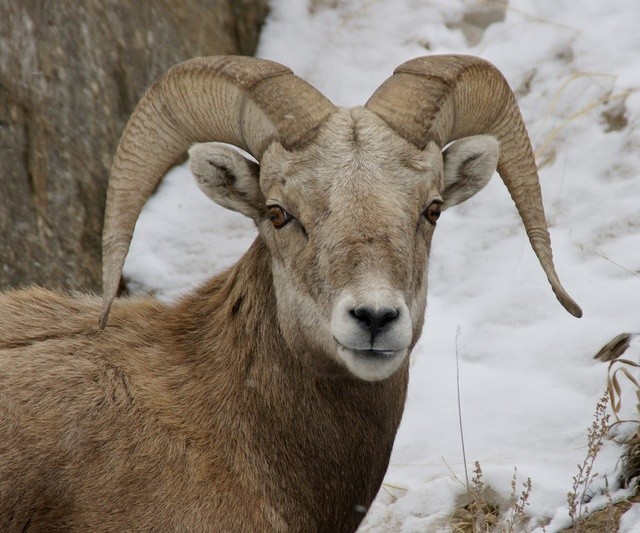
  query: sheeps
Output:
[0,54,583,533]
